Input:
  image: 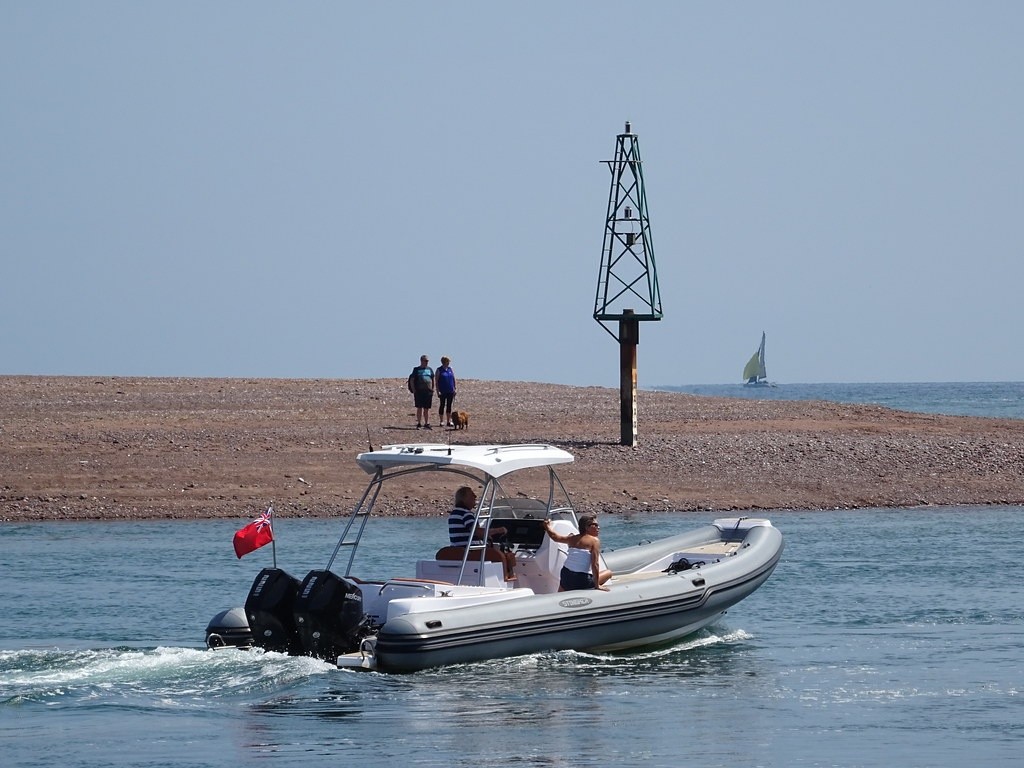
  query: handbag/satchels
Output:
[407,366,418,393]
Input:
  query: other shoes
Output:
[424,424,433,430]
[440,421,444,426]
[417,424,422,430]
[447,422,454,426]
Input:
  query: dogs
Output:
[452,411,469,430]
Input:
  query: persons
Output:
[409,355,434,430]
[435,356,457,427]
[543,512,612,592]
[448,487,507,551]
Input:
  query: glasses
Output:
[588,523,599,526]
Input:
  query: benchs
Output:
[435,544,518,581]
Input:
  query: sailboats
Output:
[742,330,778,389]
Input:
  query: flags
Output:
[233,507,273,559]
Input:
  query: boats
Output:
[204,441,785,677]
